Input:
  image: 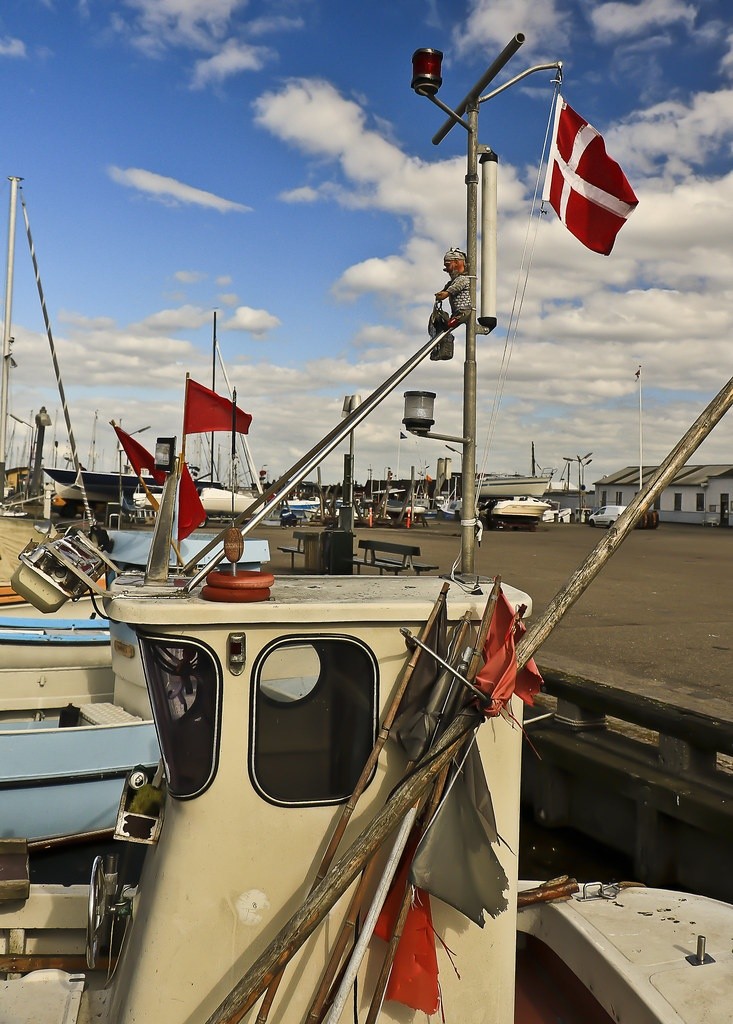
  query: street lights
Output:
[563,452,593,523]
[339,395,362,531]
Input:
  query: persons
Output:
[430,247,473,361]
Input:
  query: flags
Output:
[174,462,207,541]
[374,819,442,1015]
[114,427,168,486]
[408,728,516,930]
[474,604,528,716]
[485,589,544,705]
[184,380,252,435]
[541,92,639,256]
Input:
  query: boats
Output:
[42,311,265,518]
[0,175,110,634]
[0,372,321,861]
[0,33,733,1024]
[475,467,558,520]
[279,460,462,521]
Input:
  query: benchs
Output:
[354,540,440,576]
[277,532,304,571]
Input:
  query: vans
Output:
[589,506,628,528]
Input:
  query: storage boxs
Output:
[79,703,142,726]
